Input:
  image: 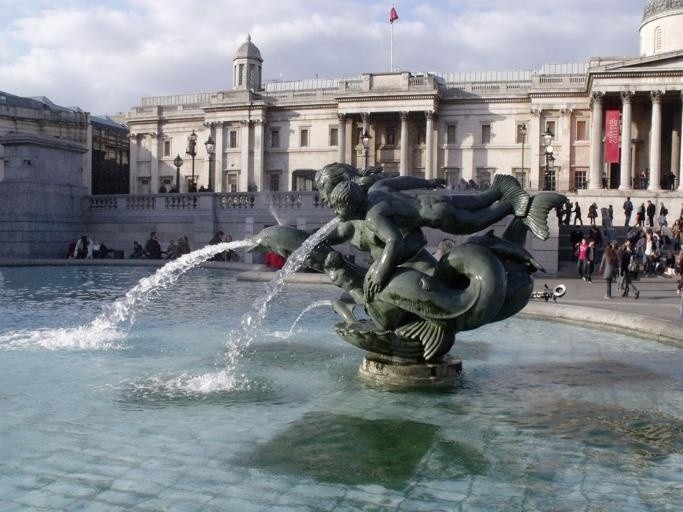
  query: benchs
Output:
[82,248,124,260]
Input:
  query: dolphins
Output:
[243,225,334,273]
[331,300,358,322]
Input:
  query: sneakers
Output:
[604,288,640,299]
[677,289,681,295]
[576,274,593,285]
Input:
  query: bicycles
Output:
[532,282,566,302]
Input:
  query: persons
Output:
[132,231,191,260]
[662,171,676,190]
[68,236,101,260]
[247,182,257,202]
[564,195,683,301]
[602,173,608,189]
[639,170,648,189]
[158,183,212,208]
[331,177,565,294]
[312,159,525,245]
[209,230,232,263]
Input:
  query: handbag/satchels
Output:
[588,212,593,217]
[663,209,668,215]
[653,249,659,258]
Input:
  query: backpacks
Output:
[628,255,639,273]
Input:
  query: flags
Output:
[388,3,399,26]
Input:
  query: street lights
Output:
[185,129,196,182]
[360,130,372,167]
[173,152,183,192]
[205,134,215,191]
[518,122,526,189]
[541,127,553,191]
